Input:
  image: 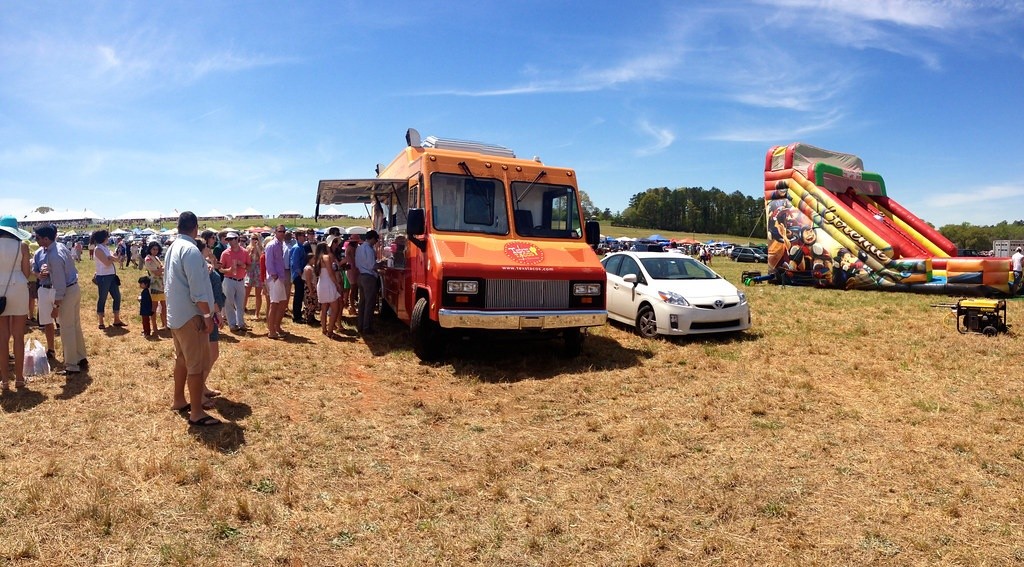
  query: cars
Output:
[599,250,752,340]
[979,249,994,256]
[700,244,735,256]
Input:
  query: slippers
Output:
[204,389,220,396]
[99,323,106,328]
[171,401,191,411]
[202,399,216,408]
[188,415,222,425]
[113,321,128,326]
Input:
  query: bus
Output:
[317,214,348,220]
[234,214,265,219]
[277,214,303,219]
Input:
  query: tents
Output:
[601,234,702,244]
[63,226,271,235]
[704,240,767,247]
[288,226,370,234]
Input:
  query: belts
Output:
[66,281,77,287]
[40,283,53,288]
[229,277,243,281]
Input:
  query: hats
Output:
[0,215,32,240]
[345,234,364,242]
[1015,247,1023,251]
[225,232,238,240]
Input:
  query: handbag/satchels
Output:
[22,338,50,375]
[341,270,350,288]
[0,296,8,315]
[114,274,121,286]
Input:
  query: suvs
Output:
[730,247,768,263]
[958,249,986,257]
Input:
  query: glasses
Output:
[240,230,315,244]
[210,236,215,239]
[227,238,235,241]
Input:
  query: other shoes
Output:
[141,307,379,338]
[0,317,88,389]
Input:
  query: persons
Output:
[115,236,126,269]
[610,242,628,252]
[93,230,129,329]
[164,211,222,426]
[683,244,711,265]
[66,237,95,262]
[0,213,90,390]
[345,230,387,334]
[144,241,170,334]
[138,276,154,335]
[194,230,252,331]
[125,240,147,269]
[249,224,352,337]
[1011,246,1024,297]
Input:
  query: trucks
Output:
[994,239,1024,258]
[315,130,609,361]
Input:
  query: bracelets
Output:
[202,313,211,318]
